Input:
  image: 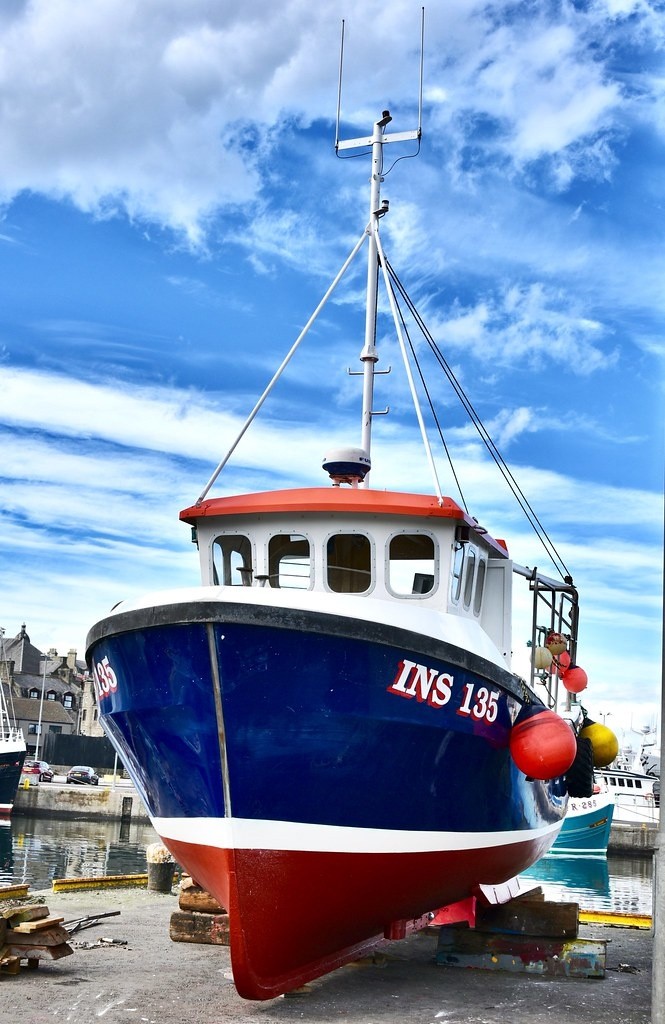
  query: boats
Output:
[82,6,617,1004]
[565,709,660,827]
[542,802,616,863]
[0,815,15,888]
[0,638,28,828]
[516,856,609,902]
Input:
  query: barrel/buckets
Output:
[146,858,176,893]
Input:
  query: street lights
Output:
[599,712,610,725]
[33,652,56,761]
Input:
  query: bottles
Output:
[537,959,543,969]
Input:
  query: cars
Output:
[66,766,99,785]
[21,759,54,783]
